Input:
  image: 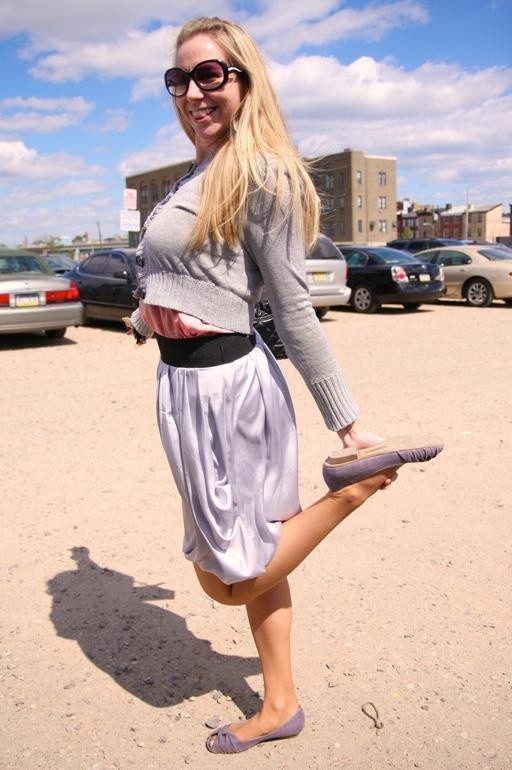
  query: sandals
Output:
[322,434,445,492]
[206,704,304,754]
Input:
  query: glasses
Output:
[164,59,245,98]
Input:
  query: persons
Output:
[122,15,441,755]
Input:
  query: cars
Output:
[0,247,148,340]
[259,232,512,320]
